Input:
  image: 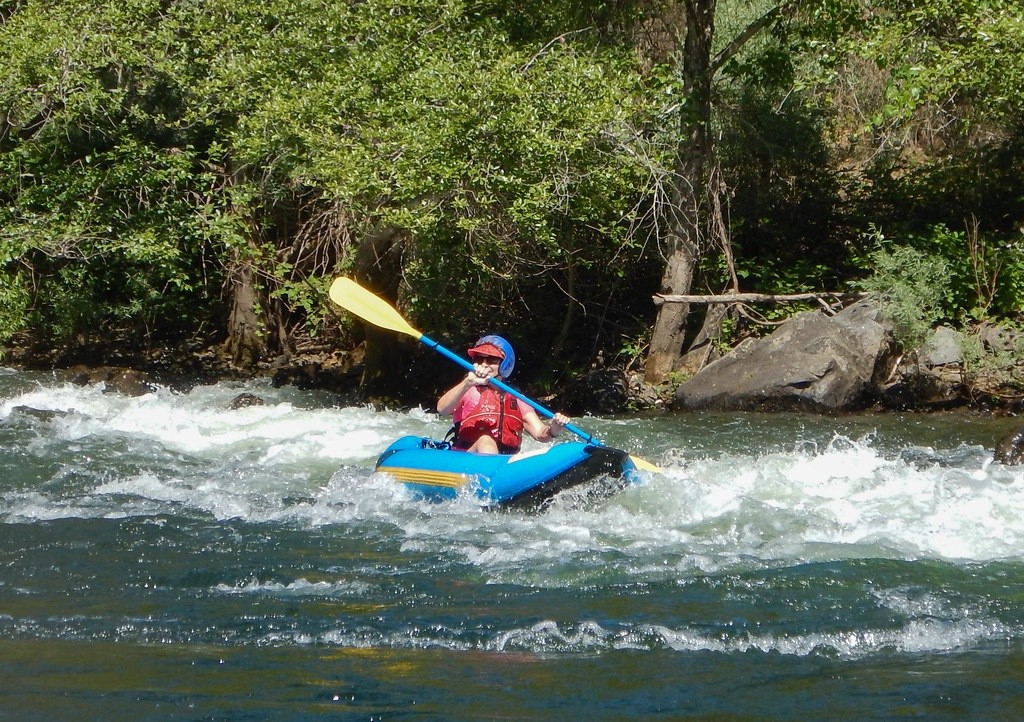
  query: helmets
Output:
[476,335,515,378]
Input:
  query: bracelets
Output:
[548,427,556,438]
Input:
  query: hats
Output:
[467,344,504,361]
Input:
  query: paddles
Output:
[328,276,665,472]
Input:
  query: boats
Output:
[375,435,641,509]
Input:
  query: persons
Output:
[436,334,572,455]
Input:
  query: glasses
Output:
[473,355,500,364]
